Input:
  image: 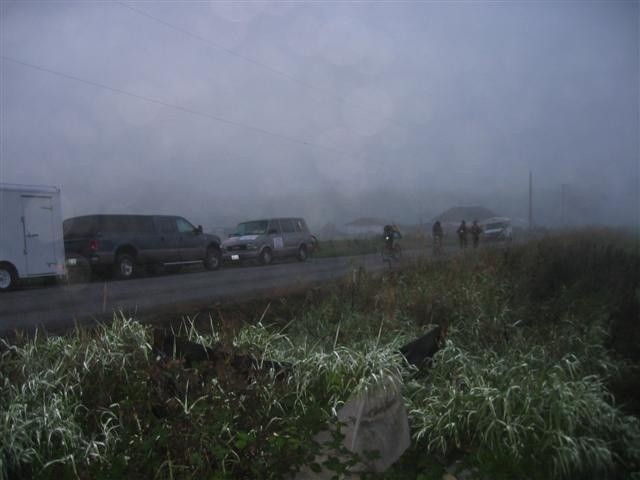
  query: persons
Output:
[469,220,483,250]
[431,220,443,247]
[456,219,468,251]
[382,223,401,254]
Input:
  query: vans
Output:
[63,214,318,279]
[484,216,513,242]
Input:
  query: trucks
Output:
[0,183,67,292]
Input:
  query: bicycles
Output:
[460,228,478,250]
[381,236,402,261]
[433,233,443,256]
